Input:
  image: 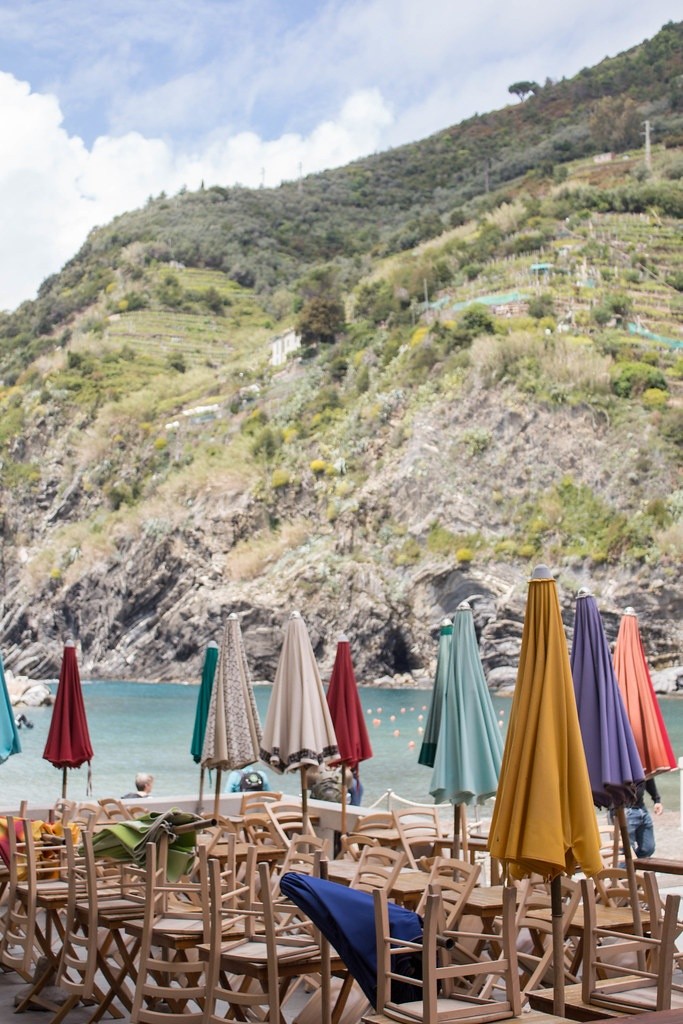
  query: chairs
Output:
[0,789,683,1024]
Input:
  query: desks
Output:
[361,1008,582,1024]
[8,882,97,1014]
[243,812,320,826]
[524,974,651,1023]
[346,871,470,912]
[51,902,149,1024]
[425,886,554,961]
[121,918,268,1014]
[349,825,448,845]
[273,861,358,888]
[511,902,652,981]
[195,934,354,1023]
[206,842,286,925]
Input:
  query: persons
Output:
[609,777,663,879]
[229,766,267,795]
[122,772,154,798]
[307,762,365,808]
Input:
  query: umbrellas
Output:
[486,565,605,1017]
[416,601,504,882]
[612,607,678,783]
[42,638,95,803]
[78,807,220,884]
[0,815,80,883]
[274,871,457,1012]
[568,587,647,972]
[193,613,263,819]
[0,647,22,765]
[324,635,372,835]
[259,611,341,855]
